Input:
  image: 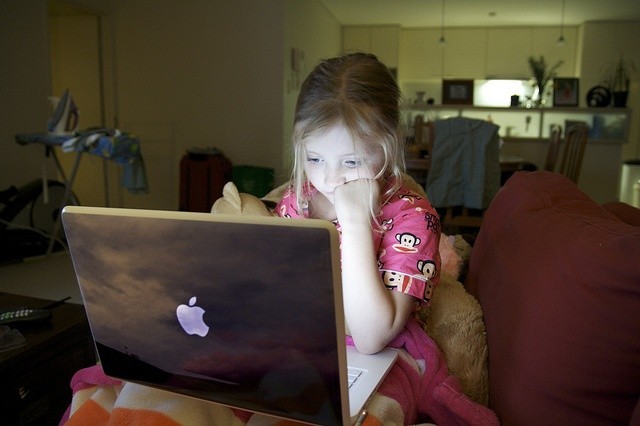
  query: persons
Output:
[273,53,442,356]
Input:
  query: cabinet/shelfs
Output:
[391,104,632,146]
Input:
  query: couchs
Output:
[58,200,640,426]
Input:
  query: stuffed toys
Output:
[211,182,273,215]
[417,232,490,407]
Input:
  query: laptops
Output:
[61,203,398,426]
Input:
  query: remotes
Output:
[3,305,53,324]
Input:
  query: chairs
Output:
[549,123,590,185]
[545,122,563,168]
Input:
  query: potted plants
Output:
[607,51,636,107]
[531,56,562,108]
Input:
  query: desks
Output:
[402,143,538,183]
[0,248,84,308]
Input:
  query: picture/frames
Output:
[441,79,473,105]
[554,77,579,106]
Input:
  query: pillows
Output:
[475,172,640,426]
